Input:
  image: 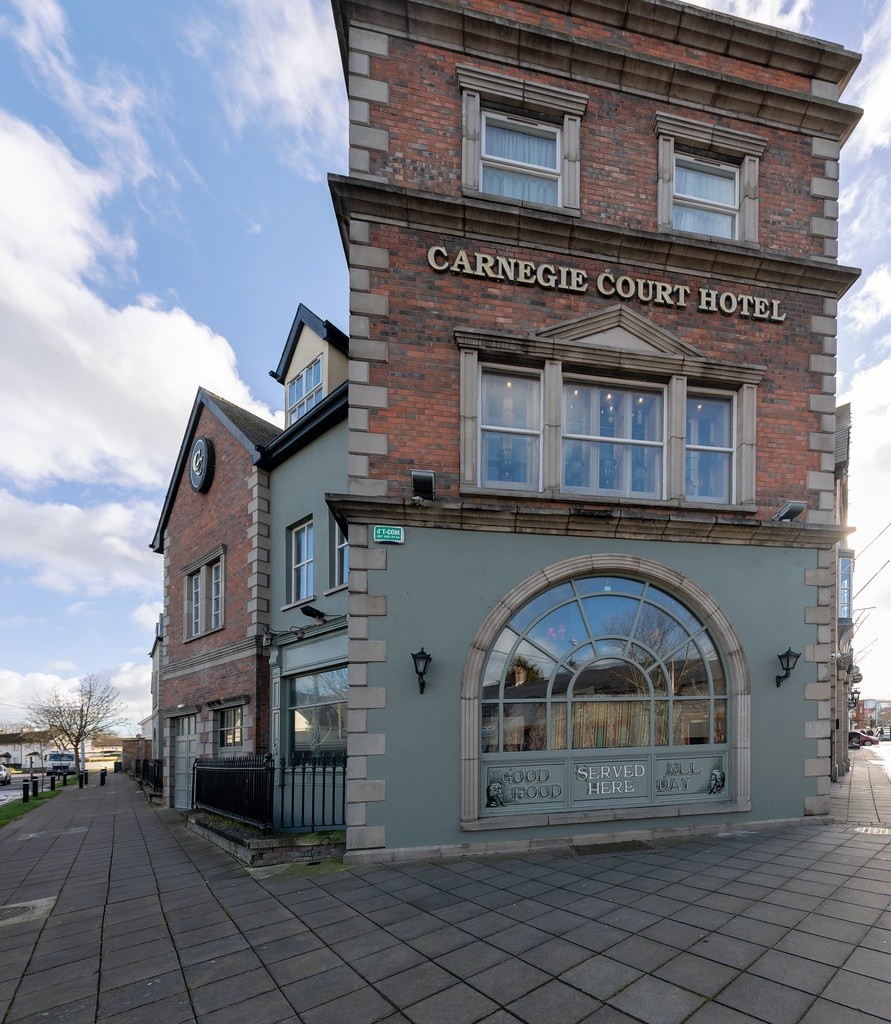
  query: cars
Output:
[849,730,879,746]
[46,752,82,774]
[0,765,11,785]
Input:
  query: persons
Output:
[861,726,884,742]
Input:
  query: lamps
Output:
[848,689,860,709]
[410,470,438,500]
[411,648,434,694]
[289,624,309,643]
[771,499,808,523]
[300,605,342,619]
[774,647,802,687]
